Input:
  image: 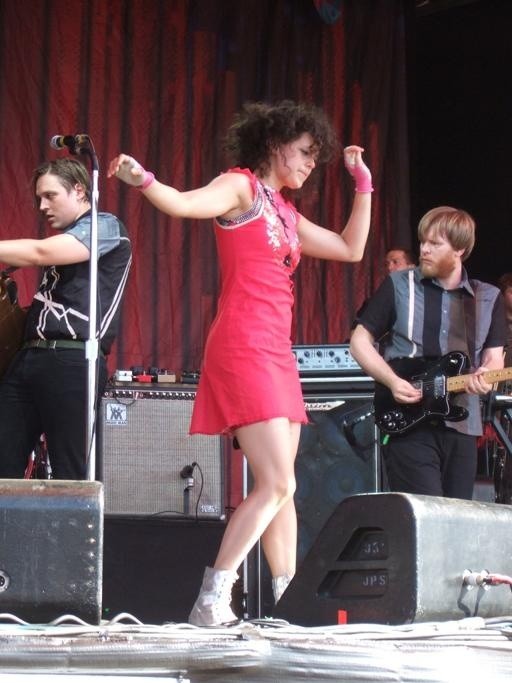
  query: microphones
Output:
[50,135,88,151]
[339,402,377,428]
[180,465,194,478]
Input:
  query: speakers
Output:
[95,387,227,522]
[0,479,105,626]
[101,519,243,626]
[243,392,380,623]
[268,492,512,628]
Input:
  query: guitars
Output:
[375,351,512,438]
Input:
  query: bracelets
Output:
[137,171,156,192]
[352,185,374,194]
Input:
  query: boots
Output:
[188,565,239,629]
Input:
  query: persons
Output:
[498,272,511,369]
[0,131,134,479]
[349,205,505,500]
[108,99,374,630]
[384,246,417,275]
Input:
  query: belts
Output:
[20,339,106,360]
